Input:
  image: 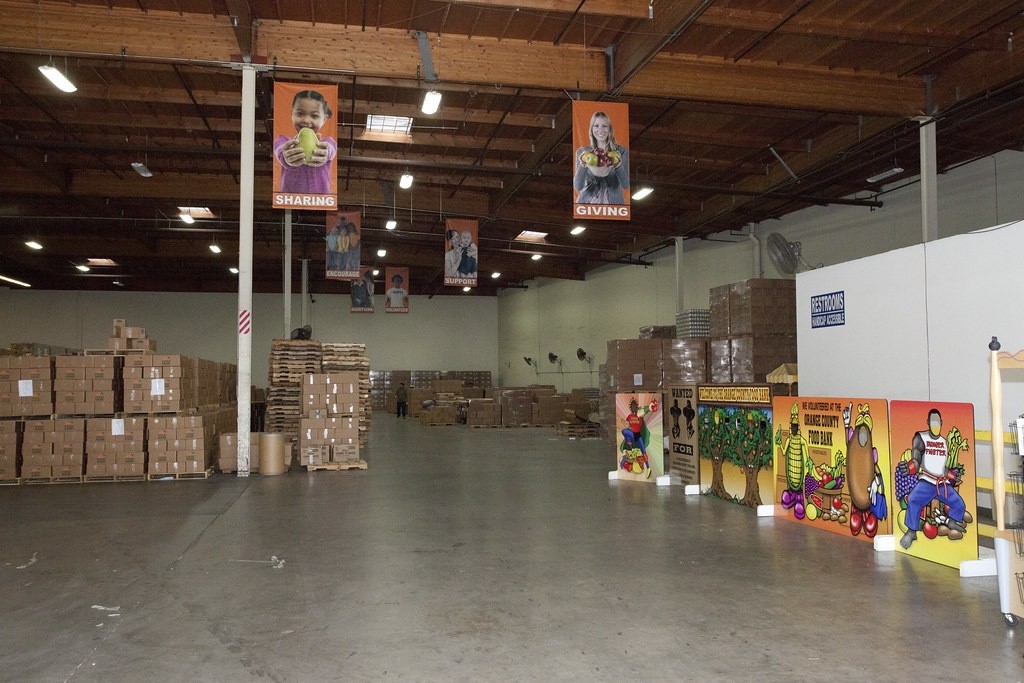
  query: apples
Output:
[581,148,621,167]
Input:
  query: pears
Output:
[292,127,320,164]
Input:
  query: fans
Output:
[524,357,536,367]
[768,231,824,274]
[577,348,590,364]
[548,352,562,366]
[291,325,312,340]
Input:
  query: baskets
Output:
[1015,572,1024,604]
[1007,474,1024,505]
[1009,423,1024,455]
[1013,528,1024,558]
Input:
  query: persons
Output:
[327,217,360,270]
[573,111,630,205]
[445,229,477,278]
[273,90,337,193]
[351,271,374,307]
[386,275,409,308]
[393,383,408,419]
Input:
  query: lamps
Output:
[131,128,153,178]
[421,30,442,115]
[377,238,387,257]
[208,207,222,254]
[386,205,397,230]
[631,163,654,201]
[38,54,78,93]
[179,175,195,224]
[398,165,413,189]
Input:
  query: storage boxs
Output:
[369,277,797,442]
[0,318,294,478]
[298,373,360,466]
